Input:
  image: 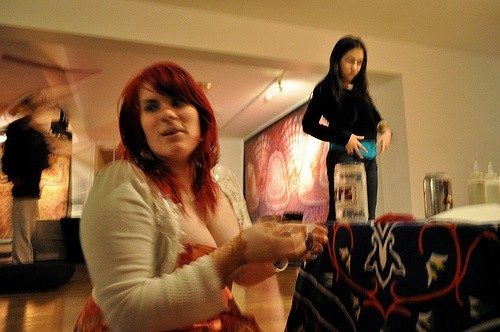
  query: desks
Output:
[283,219,499,331]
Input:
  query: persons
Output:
[72,63,328,332]
[300,37,392,222]
[1,102,49,264]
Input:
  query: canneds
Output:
[334,161,369,223]
[423,171,453,218]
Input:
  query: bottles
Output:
[484,161,500,204]
[468,162,485,205]
